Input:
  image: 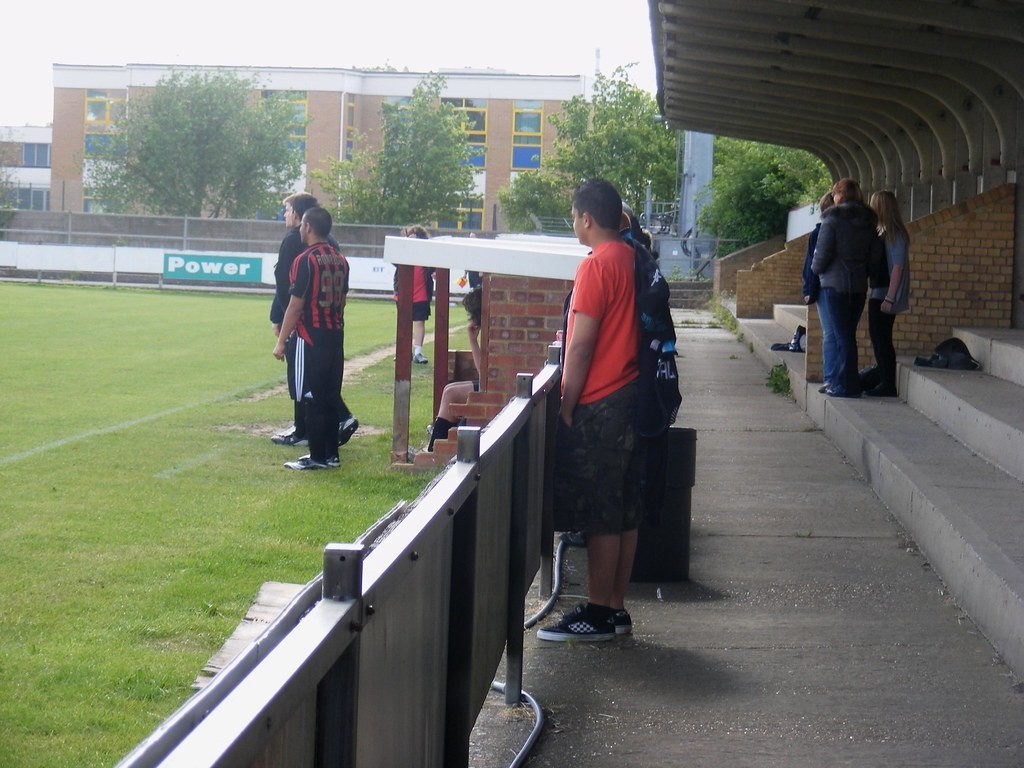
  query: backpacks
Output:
[624,236,684,438]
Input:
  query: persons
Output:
[269,193,359,471]
[802,178,911,398]
[393,224,435,363]
[463,232,483,289]
[536,177,656,643]
[407,284,483,464]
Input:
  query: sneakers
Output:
[284,457,328,470]
[337,416,359,447]
[298,454,339,467]
[564,604,632,634]
[413,353,430,364]
[537,609,616,641]
[272,431,309,446]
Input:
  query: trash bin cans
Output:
[630,426,698,582]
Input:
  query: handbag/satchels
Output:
[913,337,981,371]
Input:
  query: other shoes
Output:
[818,382,831,393]
[824,385,834,396]
[865,381,898,397]
[407,444,417,460]
[426,425,434,436]
[560,530,587,546]
